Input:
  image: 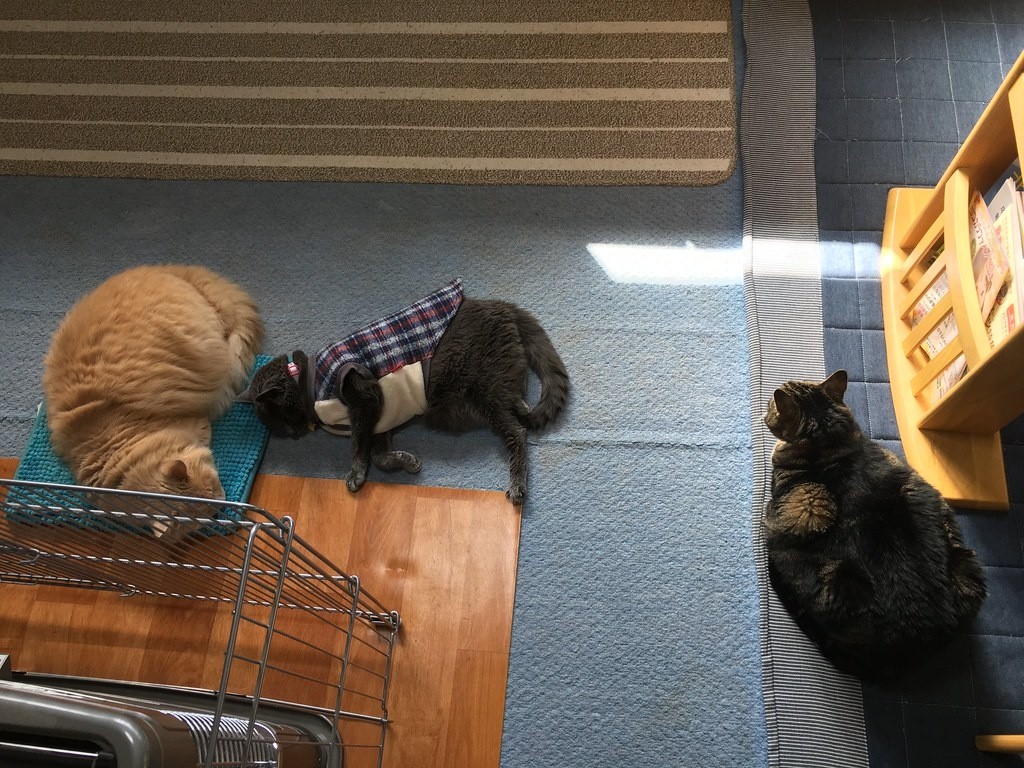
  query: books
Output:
[908,157,1024,411]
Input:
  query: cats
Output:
[232,299,571,507]
[761,368,990,678]
[41,265,263,539]
